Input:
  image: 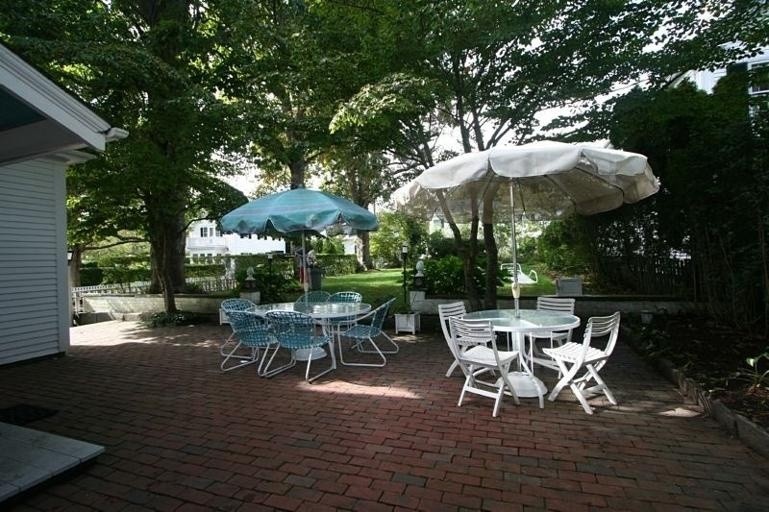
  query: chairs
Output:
[542,311,621,415]
[438,301,497,379]
[502,263,539,285]
[324,292,364,348]
[449,316,519,417]
[220,298,280,360]
[338,297,399,367]
[525,297,575,380]
[296,291,330,302]
[263,310,334,382]
[221,310,293,377]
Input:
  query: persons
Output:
[296,247,304,283]
[306,249,317,267]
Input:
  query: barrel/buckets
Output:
[640,310,653,326]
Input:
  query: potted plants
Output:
[394,303,420,335]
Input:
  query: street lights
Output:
[265,252,273,300]
[399,240,408,305]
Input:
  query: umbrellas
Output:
[386,140,661,319]
[219,184,378,308]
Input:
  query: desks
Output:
[244,302,371,369]
[462,308,580,398]
